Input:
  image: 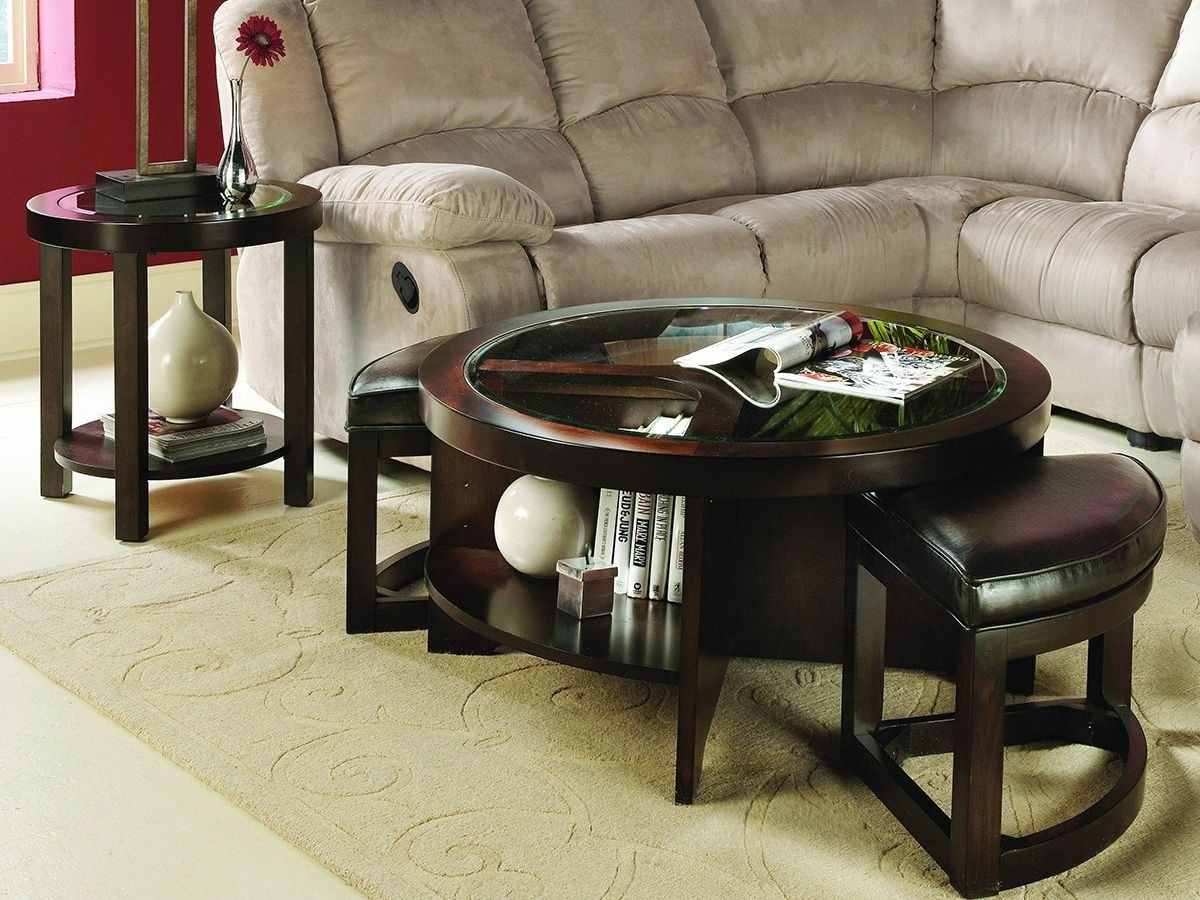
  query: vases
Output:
[147,291,237,424]
[216,78,258,203]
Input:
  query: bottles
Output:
[148,288,239,423]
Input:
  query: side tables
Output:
[25,178,322,542]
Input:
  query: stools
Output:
[839,454,1168,900]
[346,334,459,634]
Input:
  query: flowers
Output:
[235,14,287,79]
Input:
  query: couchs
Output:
[212,0,1200,539]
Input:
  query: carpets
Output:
[1,476,1200,900]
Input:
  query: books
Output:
[593,415,693,605]
[100,405,266,464]
[674,310,981,409]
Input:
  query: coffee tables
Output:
[416,298,1053,807]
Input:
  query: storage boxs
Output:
[557,543,617,620]
